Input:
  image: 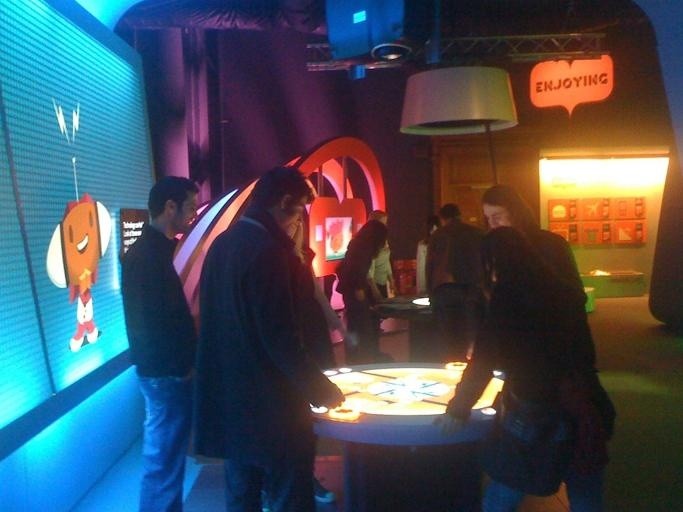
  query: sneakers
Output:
[313,479,336,502]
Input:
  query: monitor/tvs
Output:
[326,216,353,261]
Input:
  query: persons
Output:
[120,175,200,512]
[432,224,618,512]
[365,209,396,331]
[415,215,442,295]
[425,202,487,361]
[467,183,596,367]
[290,220,359,502]
[334,219,389,357]
[198,163,346,512]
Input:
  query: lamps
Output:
[399,65,519,138]
